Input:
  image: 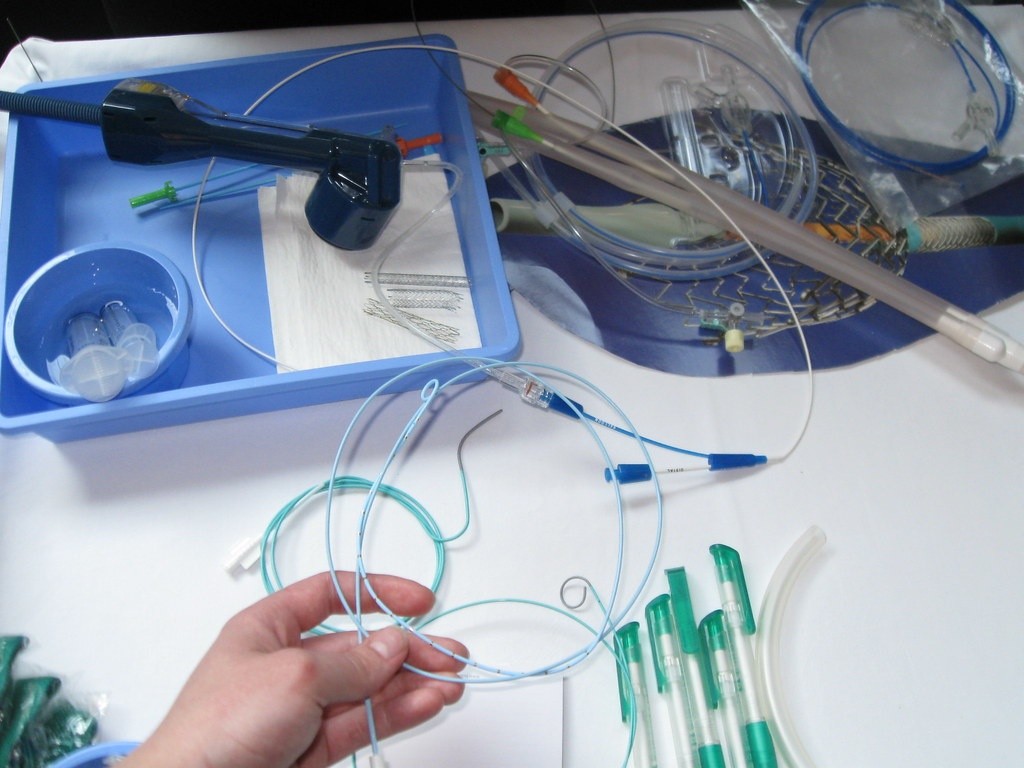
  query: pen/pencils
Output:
[645,593,703,768]
[613,621,656,768]
[710,543,778,768]
[665,565,725,768]
[698,608,755,768]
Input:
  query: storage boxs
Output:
[0,33,525,443]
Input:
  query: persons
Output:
[106,570,470,768]
